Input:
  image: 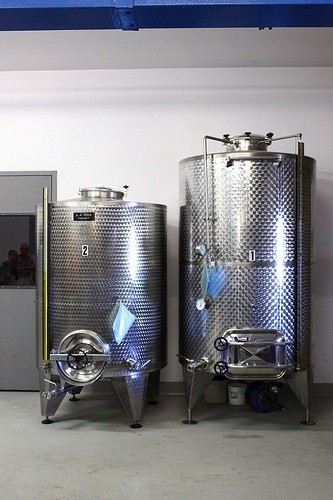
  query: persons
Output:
[0,243,37,287]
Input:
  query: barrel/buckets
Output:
[203,379,226,405]
[228,383,247,405]
